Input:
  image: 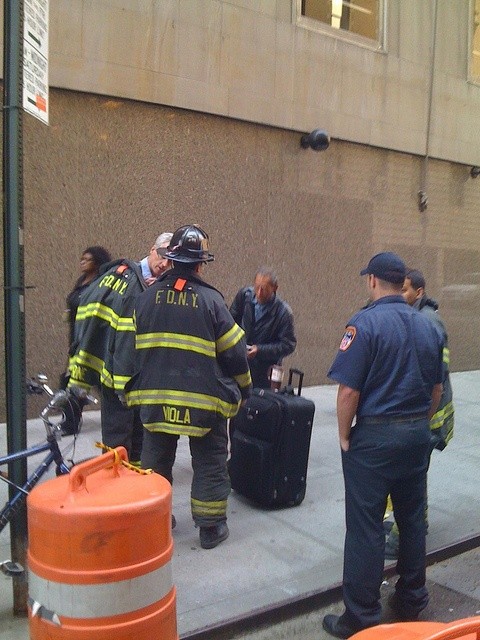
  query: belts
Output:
[356,411,429,425]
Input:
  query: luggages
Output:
[229,367,315,509]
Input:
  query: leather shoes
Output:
[171,513,177,530]
[383,541,400,558]
[198,522,229,548]
[388,592,419,622]
[321,614,357,639]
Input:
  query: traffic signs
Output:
[21,0,52,127]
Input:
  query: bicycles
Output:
[0,373,98,535]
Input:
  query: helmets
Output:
[156,224,215,264]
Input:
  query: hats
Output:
[359,252,407,283]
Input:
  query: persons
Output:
[57,245,112,436]
[128,224,254,549]
[381,269,454,558]
[99,233,175,467]
[229,266,297,388]
[321,251,443,637]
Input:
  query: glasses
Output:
[80,256,94,262]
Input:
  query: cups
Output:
[267,364,285,393]
